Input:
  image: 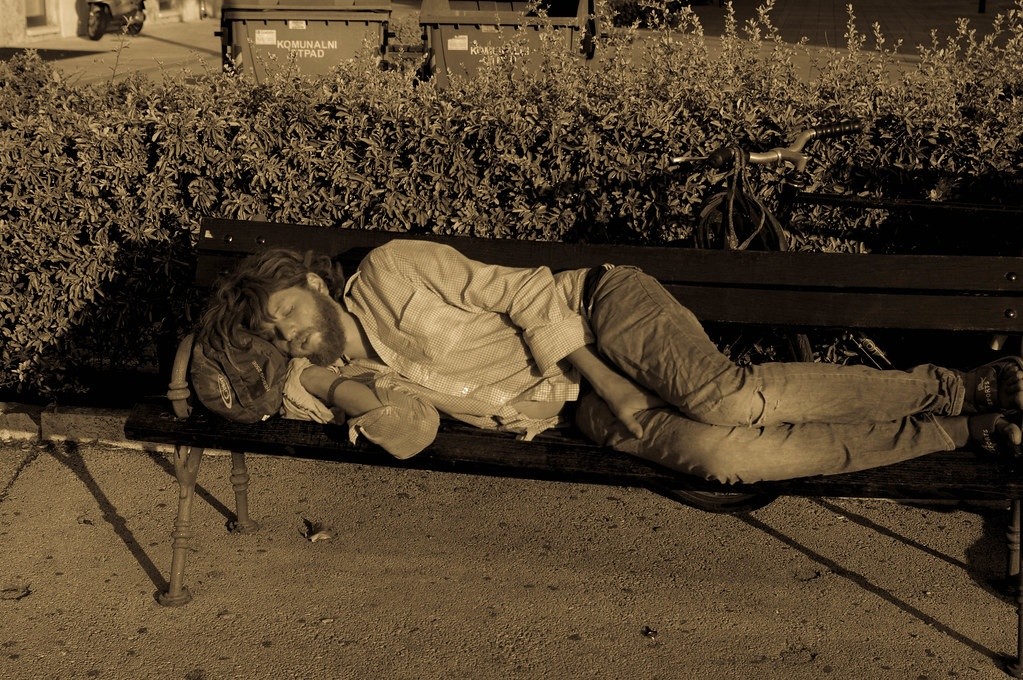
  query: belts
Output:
[583,262,615,318]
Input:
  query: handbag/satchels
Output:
[191,324,289,424]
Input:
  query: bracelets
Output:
[327,377,349,408]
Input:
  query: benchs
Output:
[124,218,1022,608]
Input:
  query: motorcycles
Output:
[87,0,145,40]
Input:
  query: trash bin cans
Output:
[219,0,392,87]
[417,0,597,92]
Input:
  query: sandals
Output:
[969,414,1023,457]
[966,355,1023,414]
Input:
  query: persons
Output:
[197,239,1023,484]
